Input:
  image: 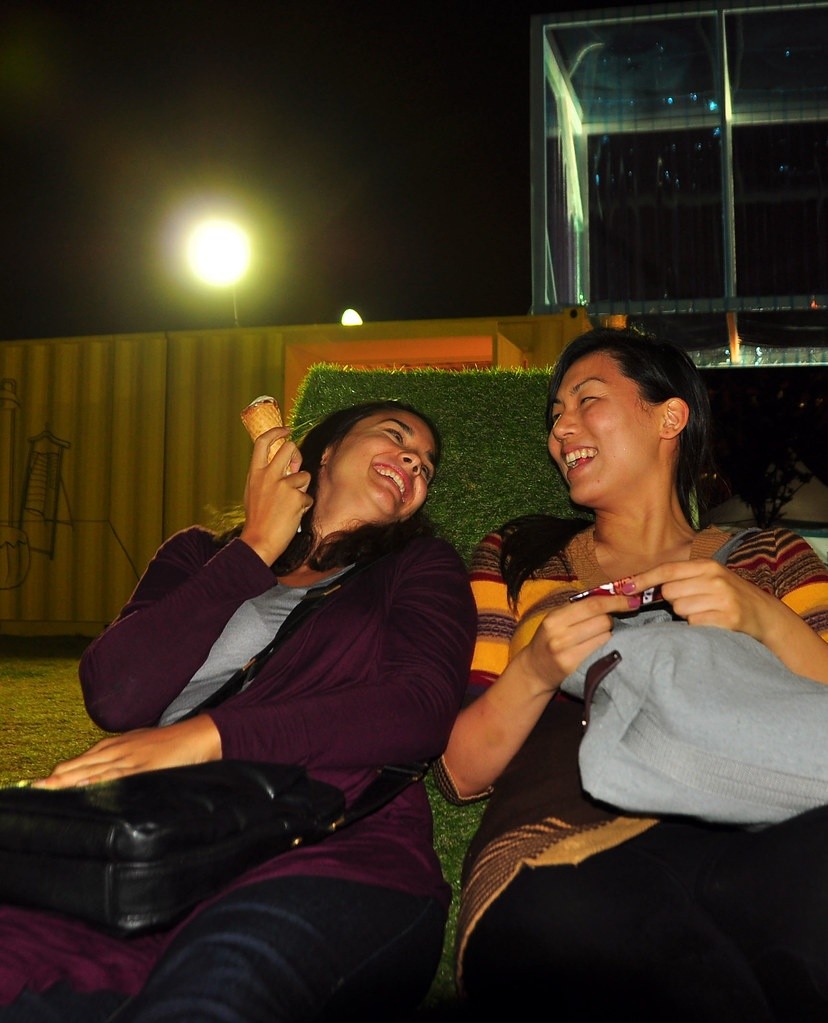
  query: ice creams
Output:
[241,395,294,477]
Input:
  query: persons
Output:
[428,328,828,1023]
[0,400,479,1023]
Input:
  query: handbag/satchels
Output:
[0,758,347,942]
[560,605,827,824]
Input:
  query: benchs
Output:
[231,366,828,1004]
